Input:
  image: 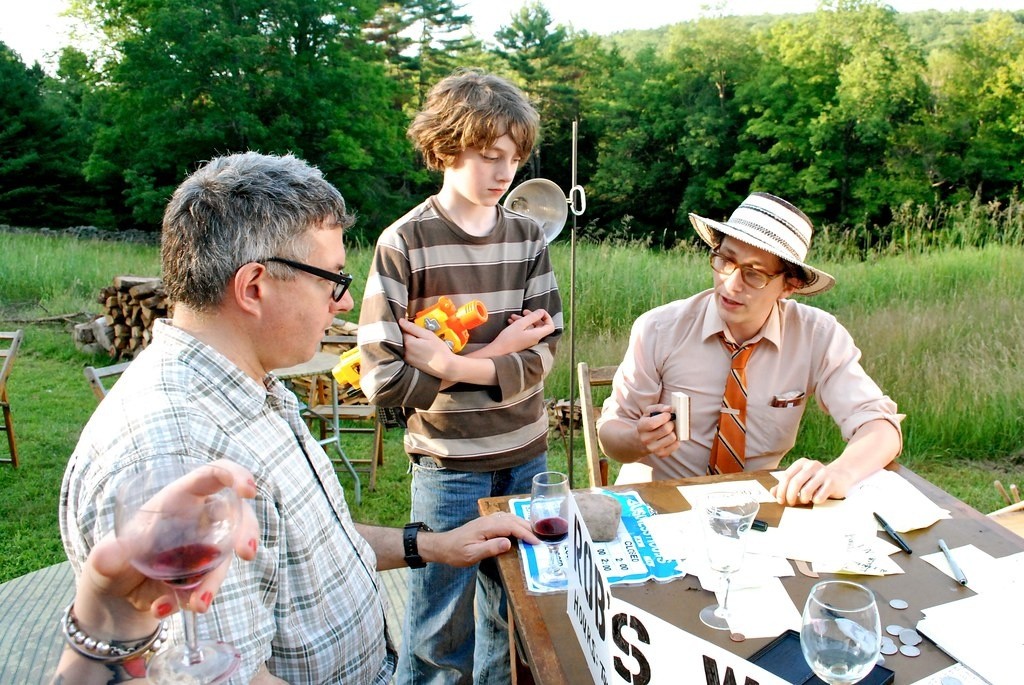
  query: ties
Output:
[707,332,765,477]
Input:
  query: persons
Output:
[59,152,539,685]
[49,458,261,685]
[357,70,563,685]
[596,192,906,506]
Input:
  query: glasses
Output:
[236,256,351,303]
[707,244,791,291]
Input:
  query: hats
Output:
[689,192,836,296]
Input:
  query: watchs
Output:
[403,522,433,569]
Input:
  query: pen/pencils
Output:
[873,512,913,554]
[938,539,969,585]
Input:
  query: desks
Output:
[270,352,362,507]
[479,459,1024,685]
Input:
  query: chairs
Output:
[577,361,635,487]
[0,329,23,470]
[84,360,132,403]
[303,330,385,491]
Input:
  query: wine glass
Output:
[697,487,759,631]
[529,471,572,591]
[113,460,241,683]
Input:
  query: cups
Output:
[799,580,881,685]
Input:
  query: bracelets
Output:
[61,598,168,662]
